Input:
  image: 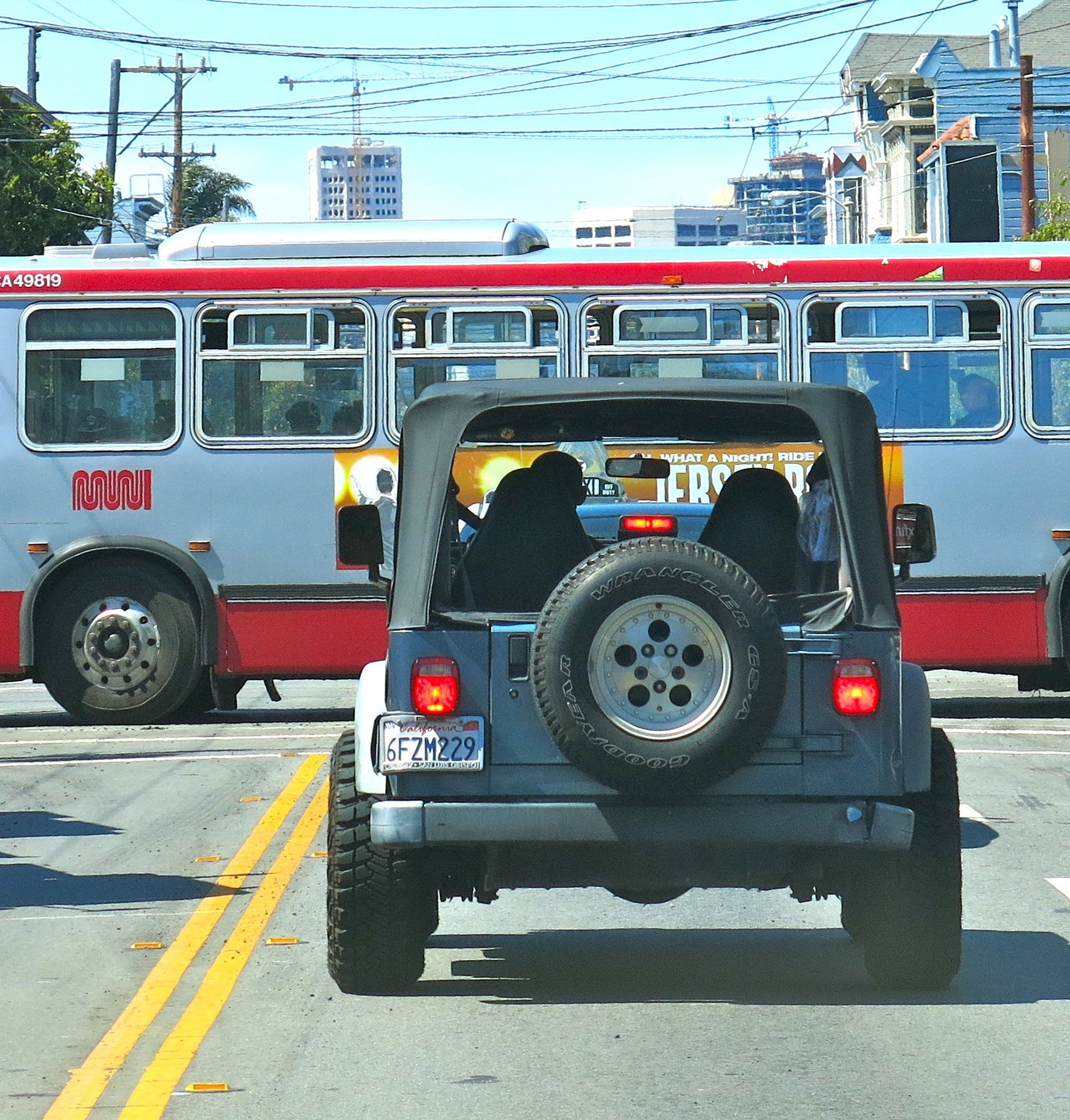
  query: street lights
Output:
[766,191,853,245]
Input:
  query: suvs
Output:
[326,378,962,990]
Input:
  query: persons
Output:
[285,401,322,435]
[529,451,602,552]
[951,374,1000,428]
[862,352,910,428]
[77,408,112,442]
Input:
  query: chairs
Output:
[698,468,839,624]
[448,468,595,612]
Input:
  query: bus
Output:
[0,218,1069,728]
